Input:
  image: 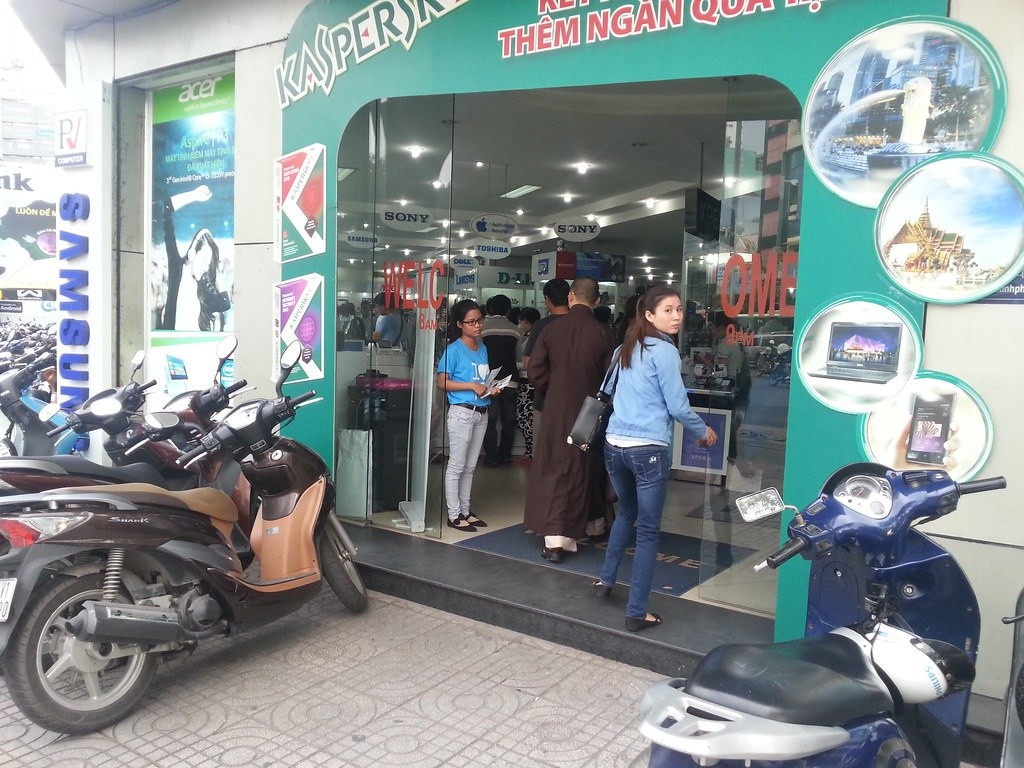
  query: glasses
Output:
[462,316,485,326]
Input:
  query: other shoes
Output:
[625,611,661,632]
[589,533,606,542]
[540,546,564,561]
[465,511,488,527]
[592,580,613,599]
[498,458,512,465]
[447,513,477,532]
[484,457,498,468]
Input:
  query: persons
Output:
[436,300,503,532]
[737,339,745,355]
[760,340,792,381]
[674,294,749,478]
[592,294,642,347]
[526,277,616,563]
[163,185,226,332]
[592,286,718,631]
[337,292,463,372]
[479,295,540,465]
[523,279,570,371]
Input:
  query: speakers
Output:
[347,401,413,512]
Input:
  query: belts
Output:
[454,403,489,414]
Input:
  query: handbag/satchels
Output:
[566,344,626,460]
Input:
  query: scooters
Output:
[0,316,261,621]
[756,351,781,376]
[637,462,1006,768]
[0,338,368,735]
[1001,589,1024,768]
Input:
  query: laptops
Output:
[805,322,903,385]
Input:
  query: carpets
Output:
[453,523,759,598]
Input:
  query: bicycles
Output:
[769,360,791,386]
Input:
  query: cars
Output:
[742,334,793,361]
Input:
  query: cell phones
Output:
[904,389,957,469]
